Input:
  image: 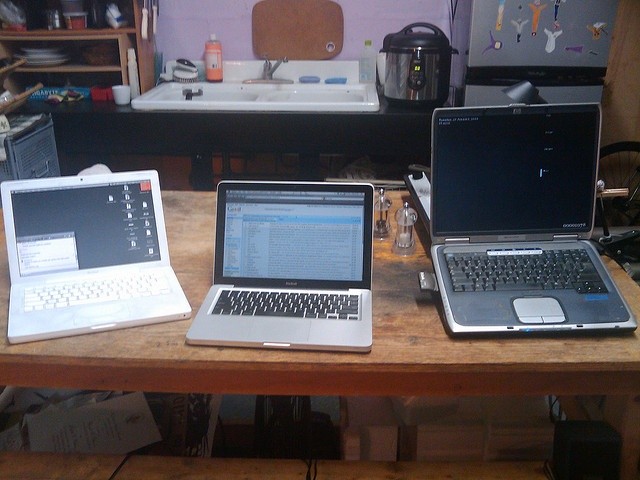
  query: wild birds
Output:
[428,101,638,338]
[0,169,193,346]
[183,179,375,354]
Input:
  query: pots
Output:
[378,23,459,108]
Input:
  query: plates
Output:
[0,0,157,96]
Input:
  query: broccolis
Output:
[452,0,617,107]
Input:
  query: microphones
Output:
[350,391,492,461]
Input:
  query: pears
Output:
[205,33,222,81]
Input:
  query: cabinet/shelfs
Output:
[204,34,223,82]
[359,40,376,83]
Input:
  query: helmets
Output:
[13,47,70,67]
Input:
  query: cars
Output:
[112,85,130,105]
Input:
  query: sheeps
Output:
[263,56,288,80]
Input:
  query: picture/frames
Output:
[0,186,640,479]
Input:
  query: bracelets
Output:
[62,0,85,12]
[63,11,89,29]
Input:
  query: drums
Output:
[141,0,149,40]
[152,0,157,35]
